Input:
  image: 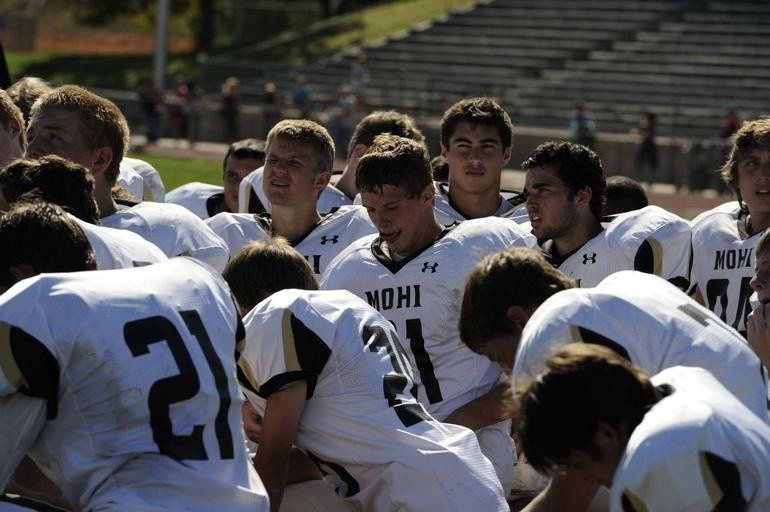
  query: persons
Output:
[0,62,768,511]
